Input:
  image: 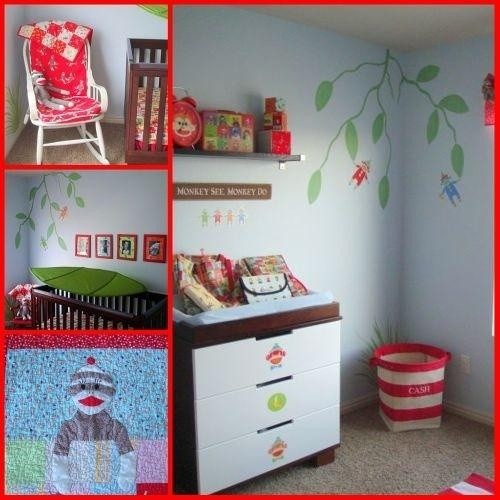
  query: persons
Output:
[122,241,130,255]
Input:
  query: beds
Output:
[32,285,167,330]
[124,39,167,164]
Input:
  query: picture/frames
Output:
[95,234,113,259]
[117,234,137,261]
[75,235,91,258]
[143,234,167,263]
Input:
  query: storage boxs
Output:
[200,109,254,154]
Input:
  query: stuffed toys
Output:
[16,298,32,322]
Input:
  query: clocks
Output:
[174,87,202,151]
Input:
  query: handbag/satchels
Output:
[239,274,292,305]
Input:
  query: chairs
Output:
[23,38,111,165]
[10,285,38,329]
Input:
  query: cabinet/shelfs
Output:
[173,300,341,495]
